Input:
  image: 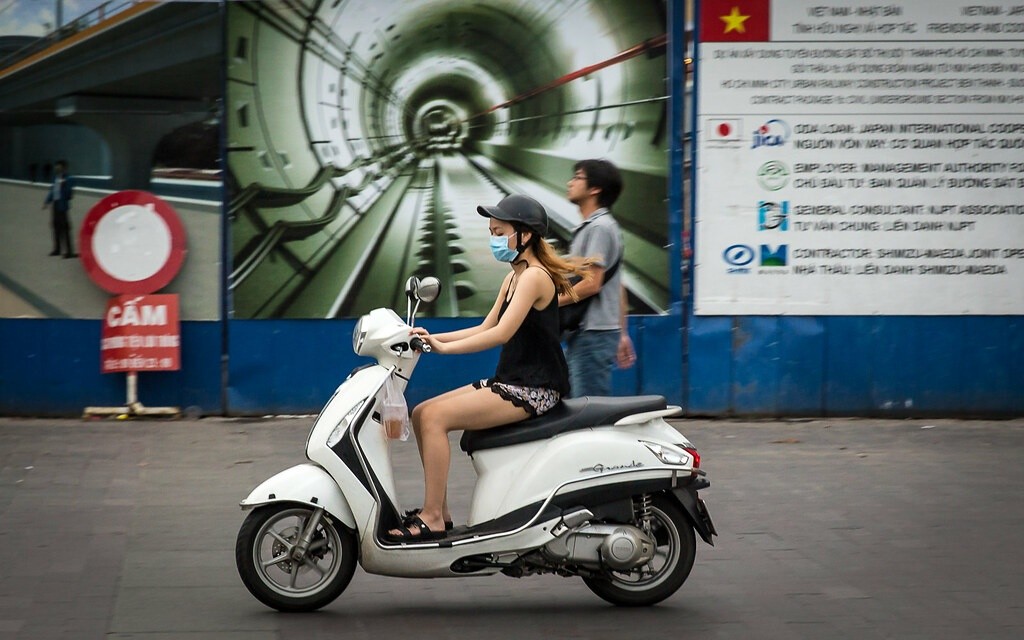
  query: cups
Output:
[383,404,405,438]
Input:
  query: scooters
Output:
[233,276,719,612]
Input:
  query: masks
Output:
[490,232,520,262]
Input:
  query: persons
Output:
[385,194,605,542]
[41,159,76,259]
[553,160,637,398]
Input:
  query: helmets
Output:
[477,194,547,238]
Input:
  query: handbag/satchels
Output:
[558,276,593,331]
[381,374,410,441]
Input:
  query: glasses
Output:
[572,175,588,182]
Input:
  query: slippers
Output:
[386,515,448,542]
[401,508,453,530]
